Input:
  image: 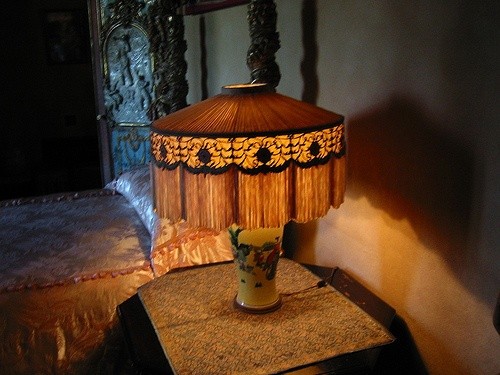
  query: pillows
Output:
[107,166,235,276]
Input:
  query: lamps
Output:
[143,85,347,313]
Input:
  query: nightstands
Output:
[117,261,395,375]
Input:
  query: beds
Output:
[2,1,281,369]
